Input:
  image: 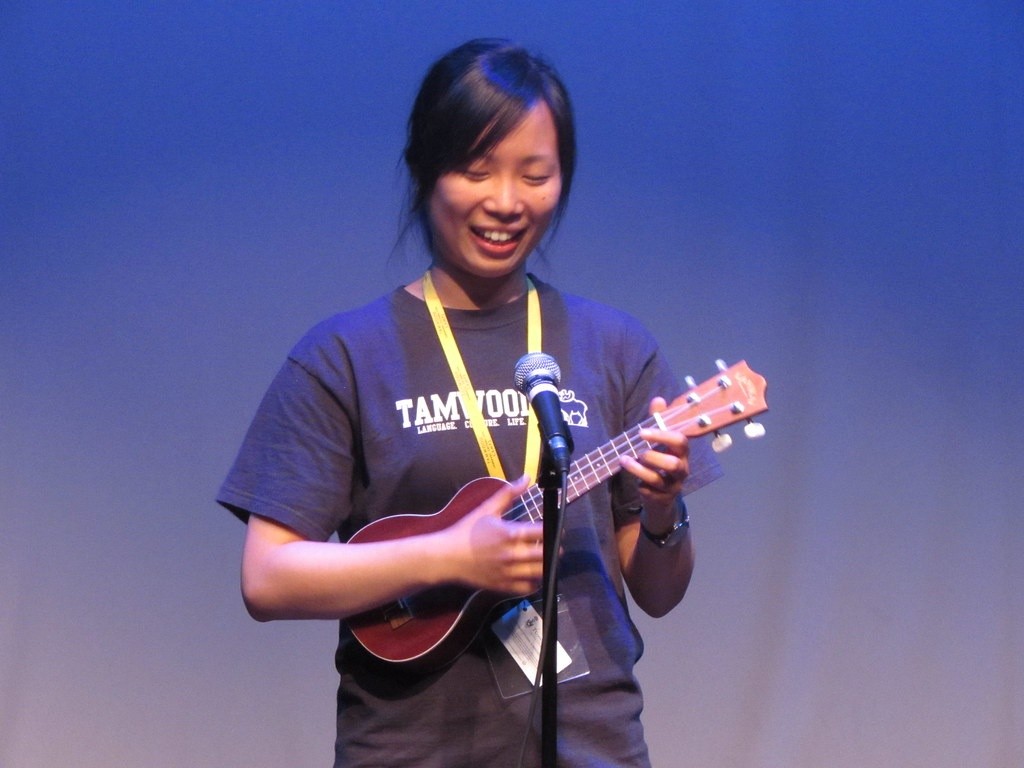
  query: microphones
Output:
[513,352,571,476]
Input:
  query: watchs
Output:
[639,498,691,550]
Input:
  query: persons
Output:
[215,37,694,767]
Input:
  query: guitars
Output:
[344,356,771,674]
